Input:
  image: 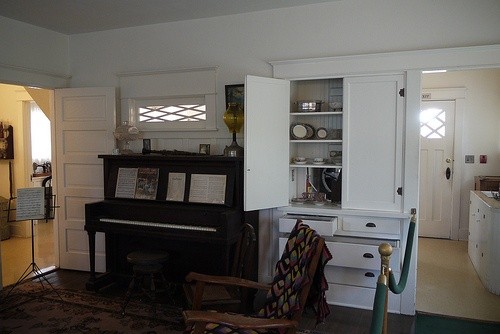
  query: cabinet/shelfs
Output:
[467,190,500,297]
[239,56,411,313]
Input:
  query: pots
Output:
[295,100,321,111]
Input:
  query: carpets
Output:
[0,279,319,334]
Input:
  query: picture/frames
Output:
[225,83,245,111]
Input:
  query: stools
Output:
[120,248,183,325]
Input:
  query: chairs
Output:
[186,222,257,310]
[182,218,325,334]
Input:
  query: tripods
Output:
[2,194,65,306]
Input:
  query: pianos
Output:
[84,154,259,291]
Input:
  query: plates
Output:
[314,201,325,206]
[290,122,328,140]
[294,161,307,164]
[312,162,326,165]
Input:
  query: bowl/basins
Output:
[313,158,323,162]
[295,157,306,162]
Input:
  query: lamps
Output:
[113,120,139,154]
[223,102,244,157]
